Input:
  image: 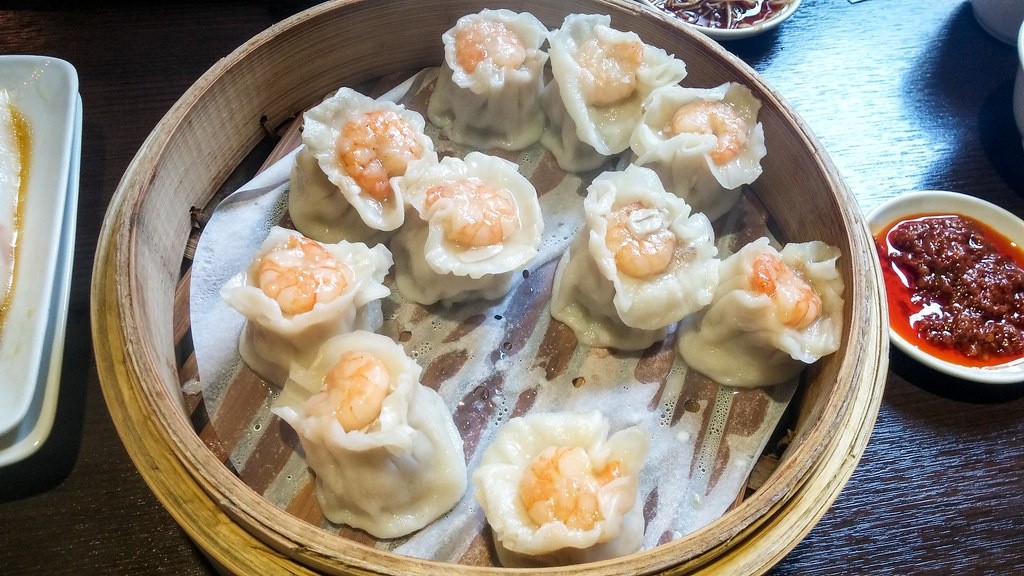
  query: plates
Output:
[864,191,1024,384]
[637,0,804,40]
[0,55,82,468]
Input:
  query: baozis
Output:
[218,7,846,567]
